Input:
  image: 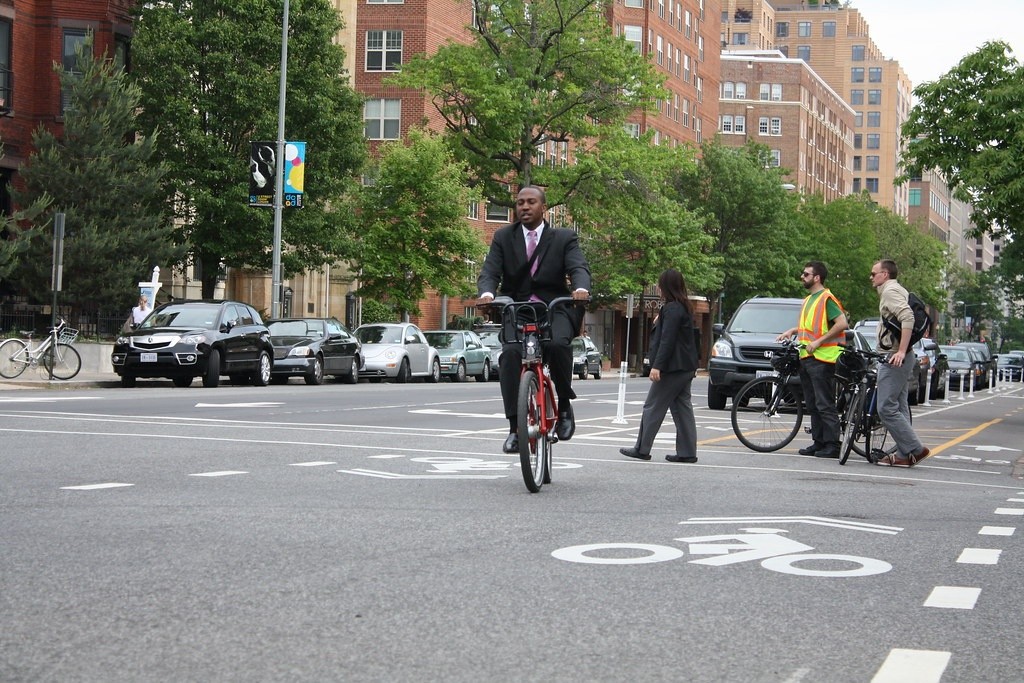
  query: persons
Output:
[869,259,931,468]
[775,261,849,459]
[619,269,699,463]
[131,294,156,328]
[582,331,591,339]
[476,185,591,454]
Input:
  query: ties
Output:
[528,230,539,301]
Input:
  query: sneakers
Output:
[909,446,930,467]
[877,454,910,468]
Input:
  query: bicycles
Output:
[729,338,913,459]
[0,317,82,380]
[837,342,887,464]
[476,293,594,492]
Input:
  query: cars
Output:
[938,345,981,391]
[472,328,502,382]
[997,354,1024,382]
[970,349,991,390]
[421,330,492,382]
[921,337,950,401]
[263,315,365,386]
[111,299,275,388]
[572,335,602,379]
[352,322,442,383]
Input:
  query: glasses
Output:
[802,271,818,277]
[870,271,886,277]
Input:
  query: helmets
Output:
[835,352,867,382]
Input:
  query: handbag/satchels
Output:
[694,327,703,359]
[491,282,516,328]
[123,312,133,333]
[882,292,931,352]
[775,349,800,370]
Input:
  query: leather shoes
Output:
[556,403,575,441]
[665,455,698,463]
[799,442,823,456]
[502,432,519,453]
[619,448,651,461]
[815,441,842,458]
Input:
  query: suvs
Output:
[706,295,805,410]
[854,318,937,406]
[954,342,999,389]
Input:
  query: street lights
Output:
[964,302,987,342]
